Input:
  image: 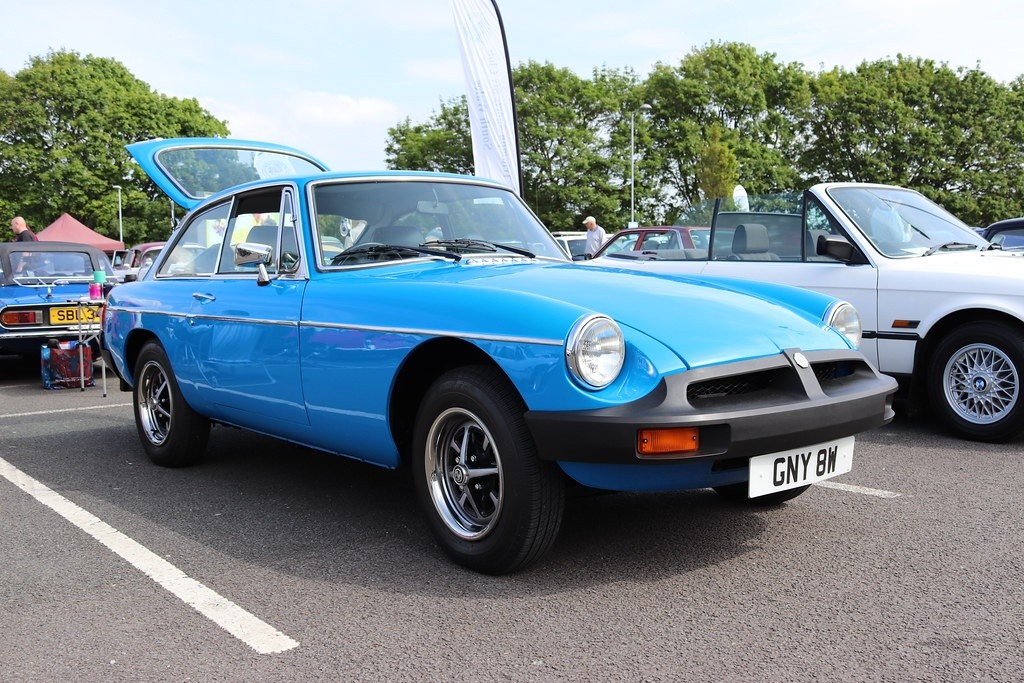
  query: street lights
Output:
[627,103,653,230]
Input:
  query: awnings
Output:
[34,213,125,276]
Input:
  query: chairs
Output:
[806,230,842,263]
[237,225,298,264]
[182,243,237,273]
[370,226,429,257]
[729,224,782,263]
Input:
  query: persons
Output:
[8,215,34,241]
[582,216,608,260]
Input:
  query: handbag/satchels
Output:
[40,340,96,389]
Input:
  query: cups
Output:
[103,282,115,300]
[89,283,102,300]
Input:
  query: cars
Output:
[97,133,899,577]
[576,180,1023,442]
[0,216,1023,365]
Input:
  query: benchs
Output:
[611,248,709,261]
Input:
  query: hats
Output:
[582,216,596,225]
